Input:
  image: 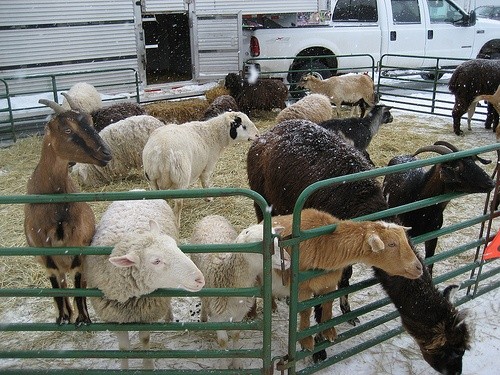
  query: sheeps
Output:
[22,56,500,375]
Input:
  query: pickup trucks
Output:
[242,0,500,102]
